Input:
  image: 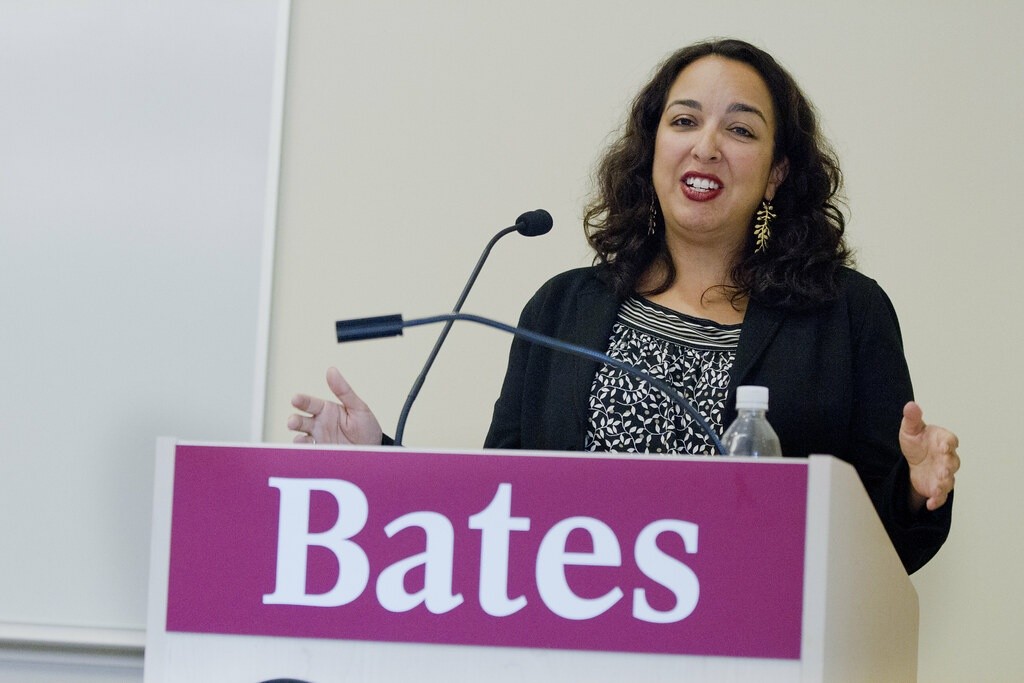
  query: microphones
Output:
[396,208,554,446]
[336,312,728,457]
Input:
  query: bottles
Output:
[720,385,782,458]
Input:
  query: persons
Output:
[284,37,963,576]
